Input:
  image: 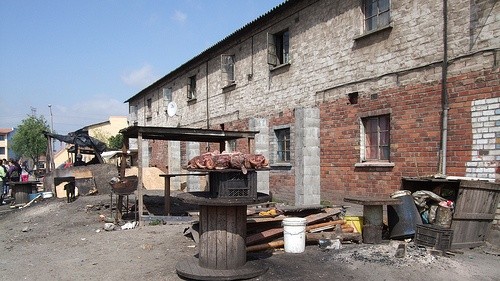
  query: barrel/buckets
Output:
[29,194,36,201]
[281,217,307,253]
[390,190,410,199]
[343,216,363,235]
[386,196,425,239]
[436,205,453,228]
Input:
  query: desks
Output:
[176,192,269,281]
[6,182,41,204]
[159,172,210,214]
[343,197,403,244]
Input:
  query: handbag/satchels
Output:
[9,167,19,178]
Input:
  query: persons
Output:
[74,154,87,166]
[0,158,22,204]
[64,158,73,168]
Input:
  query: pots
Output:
[110,179,137,193]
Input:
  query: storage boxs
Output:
[208,171,257,200]
[415,224,455,250]
